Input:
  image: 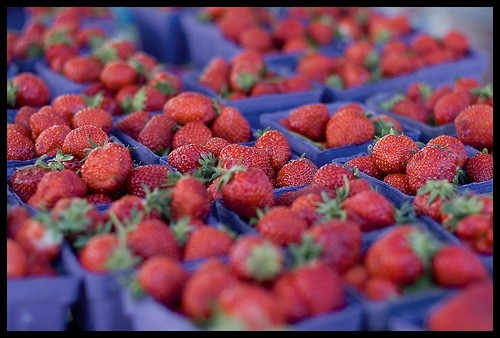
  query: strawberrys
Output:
[6,6,494,334]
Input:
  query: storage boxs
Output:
[7,7,492,331]
